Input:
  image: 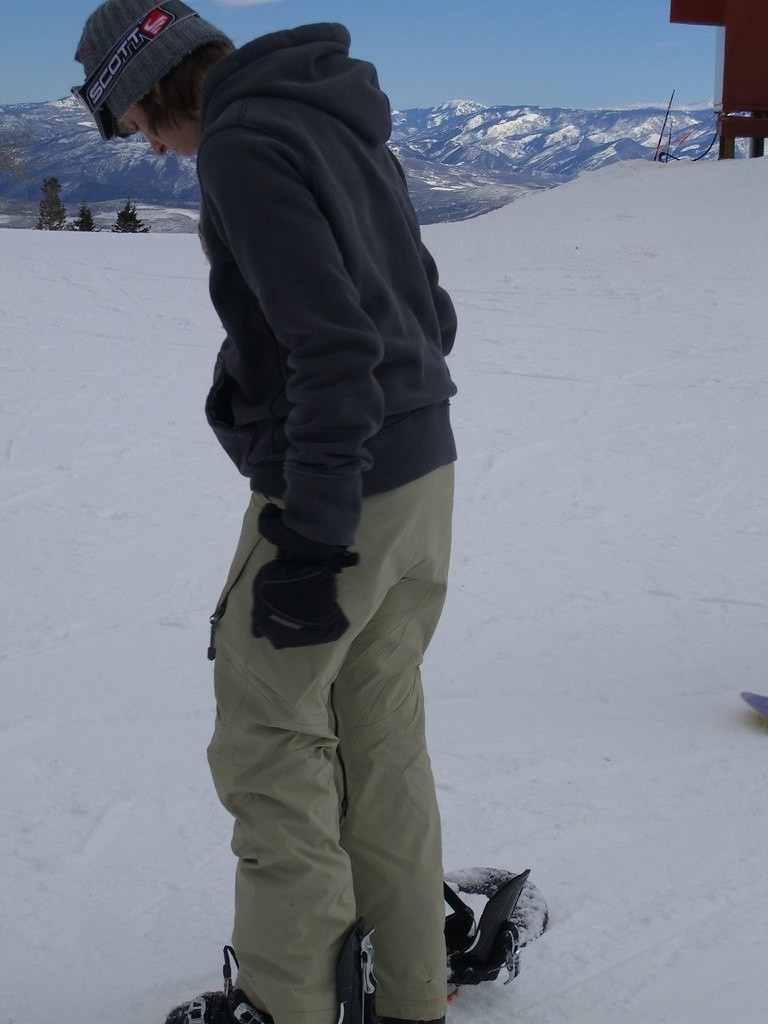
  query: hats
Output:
[70,0,237,142]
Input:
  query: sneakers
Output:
[162,988,274,1024]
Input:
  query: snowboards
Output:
[441,866,549,951]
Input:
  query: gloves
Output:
[248,526,360,649]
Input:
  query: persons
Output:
[71,0,457,1024]
